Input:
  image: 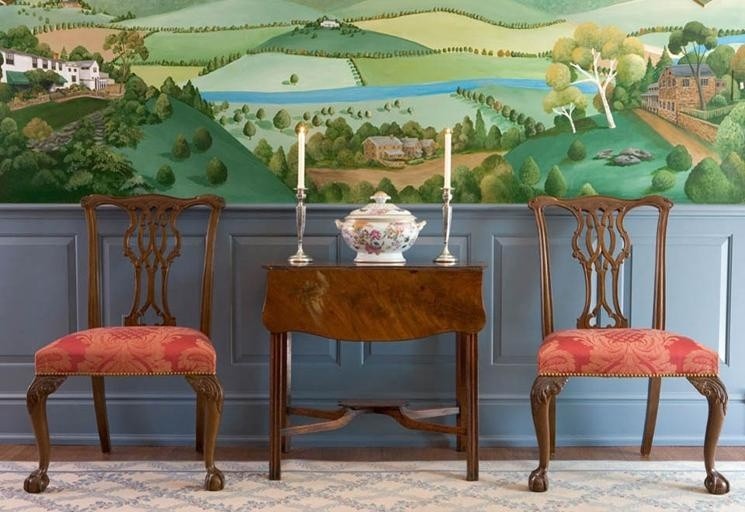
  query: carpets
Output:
[0,461,745,512]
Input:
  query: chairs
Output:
[23,192,226,492]
[521,194,730,495]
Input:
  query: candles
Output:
[297,126,306,188]
[443,125,452,188]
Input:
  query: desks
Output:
[263,262,485,481]
